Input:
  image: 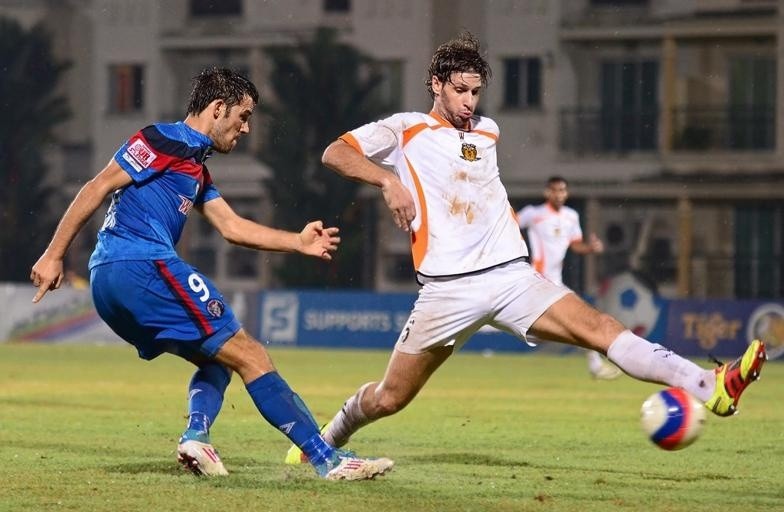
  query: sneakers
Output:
[325,456,395,485]
[285,423,339,466]
[176,430,228,478]
[705,338,768,418]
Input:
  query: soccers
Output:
[641,388,706,452]
[594,268,659,338]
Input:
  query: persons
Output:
[284,42,766,464]
[506,177,624,379]
[28,67,392,485]
